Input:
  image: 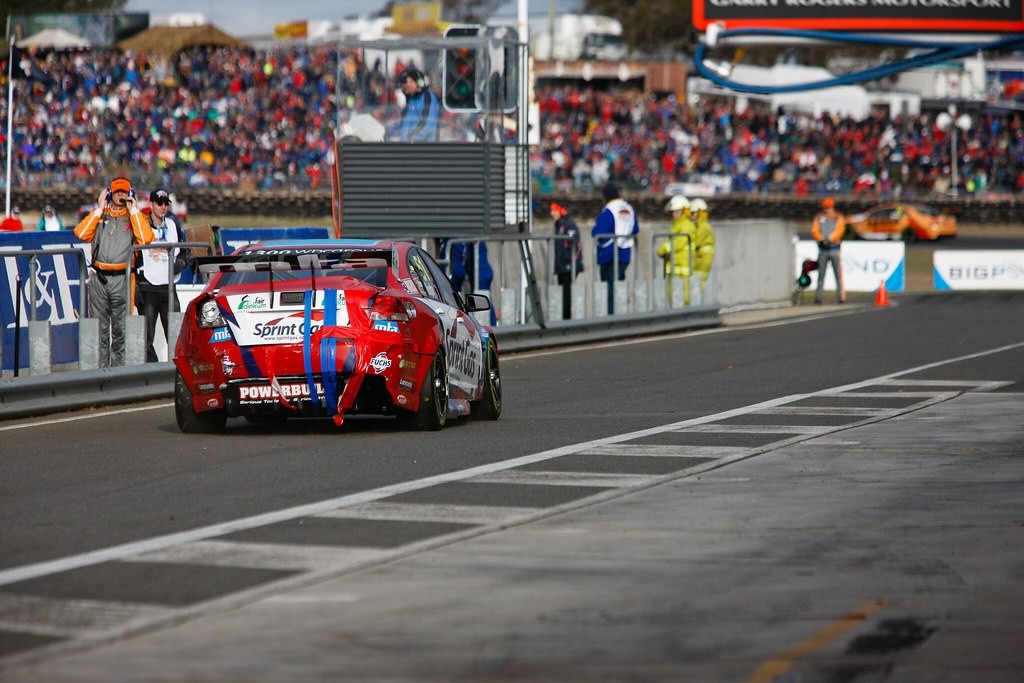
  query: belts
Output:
[97,269,126,276]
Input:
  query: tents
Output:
[16,28,90,49]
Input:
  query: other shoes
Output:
[814,299,821,304]
[839,300,845,303]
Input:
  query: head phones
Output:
[106,177,136,200]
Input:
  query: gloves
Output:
[174,260,185,275]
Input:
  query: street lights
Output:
[936,103,972,195]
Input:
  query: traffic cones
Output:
[871,276,891,307]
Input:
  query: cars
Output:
[76,193,188,224]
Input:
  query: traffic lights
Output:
[442,23,483,115]
[797,259,819,289]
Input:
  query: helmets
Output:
[690,198,708,213]
[670,194,690,210]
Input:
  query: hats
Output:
[149,189,172,204]
[110,177,131,193]
[821,197,836,208]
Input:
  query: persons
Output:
[134,189,190,362]
[0,45,1024,197]
[36,204,64,231]
[656,195,695,309]
[811,196,846,303]
[74,177,153,369]
[591,182,639,283]
[550,201,584,320]
[386,69,440,144]
[0,206,23,232]
[439,238,496,327]
[691,198,716,291]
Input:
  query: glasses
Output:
[153,200,170,207]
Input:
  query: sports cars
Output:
[172,238,502,431]
[841,202,957,244]
